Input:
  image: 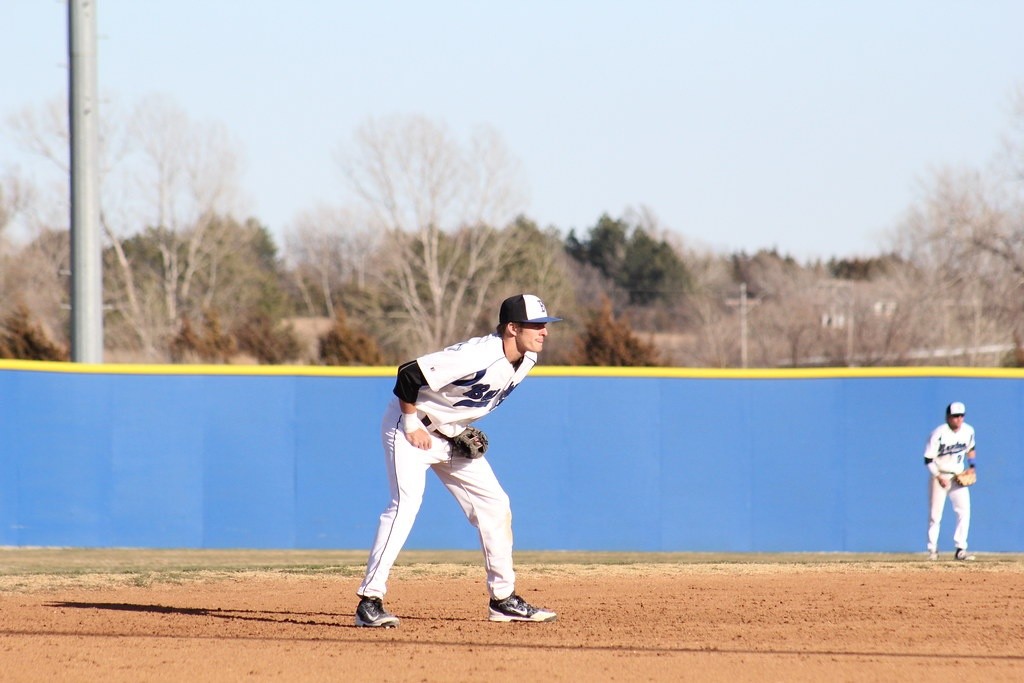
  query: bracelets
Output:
[968,460,975,464]
[970,464,974,467]
[401,412,421,433]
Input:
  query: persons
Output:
[924,402,975,561]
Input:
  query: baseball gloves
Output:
[953,470,976,486]
[453,426,489,460]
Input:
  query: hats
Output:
[500,294,564,323]
[947,401,965,415]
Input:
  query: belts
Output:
[421,415,454,442]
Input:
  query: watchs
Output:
[355,294,562,628]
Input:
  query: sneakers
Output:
[355,596,399,628]
[928,552,938,561]
[489,593,556,622]
[955,548,975,561]
